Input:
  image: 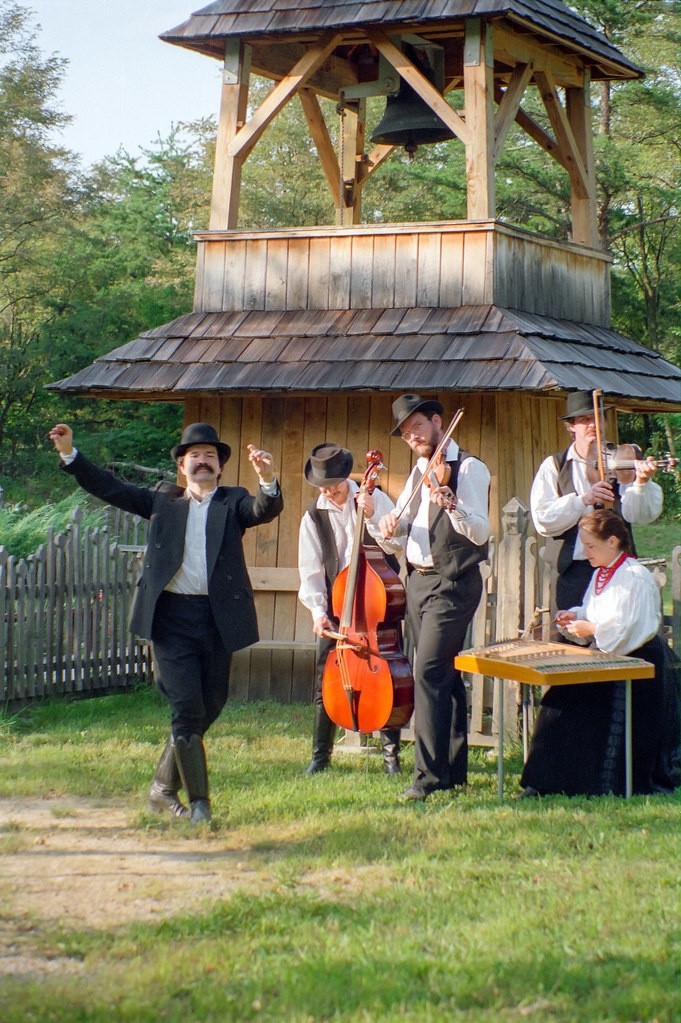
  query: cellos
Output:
[321,449,415,735]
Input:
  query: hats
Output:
[389,394,445,437]
[557,389,616,422]
[170,423,231,466]
[303,442,354,487]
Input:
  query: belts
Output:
[415,569,439,576]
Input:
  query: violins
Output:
[585,437,680,489]
[416,441,458,512]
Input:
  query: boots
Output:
[381,725,404,781]
[173,734,216,831]
[150,734,190,819]
[297,707,337,780]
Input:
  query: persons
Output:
[49,423,285,829]
[378,393,489,804]
[298,442,409,776]
[530,388,663,632]
[520,509,681,797]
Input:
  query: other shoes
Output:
[510,786,537,802]
[403,788,427,802]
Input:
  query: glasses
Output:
[401,417,428,440]
